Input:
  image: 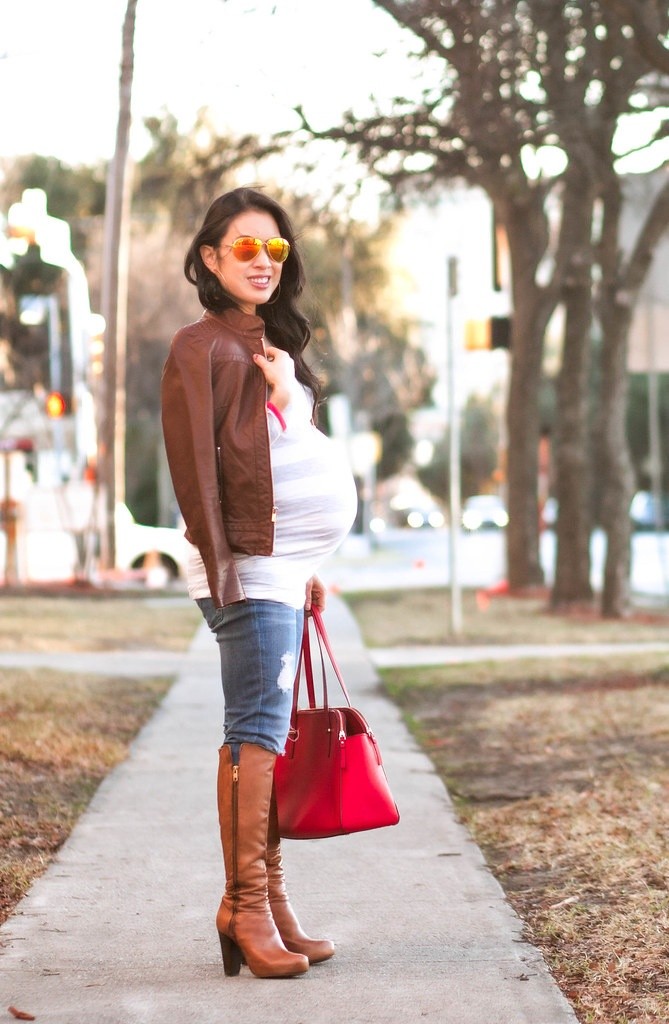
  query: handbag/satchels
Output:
[273,606,400,840]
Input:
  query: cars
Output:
[373,478,509,529]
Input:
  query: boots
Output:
[266,783,333,964]
[215,743,311,977]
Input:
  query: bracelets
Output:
[267,401,287,432]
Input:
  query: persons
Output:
[162,187,358,983]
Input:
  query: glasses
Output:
[217,233,291,264]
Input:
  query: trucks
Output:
[1,186,108,578]
